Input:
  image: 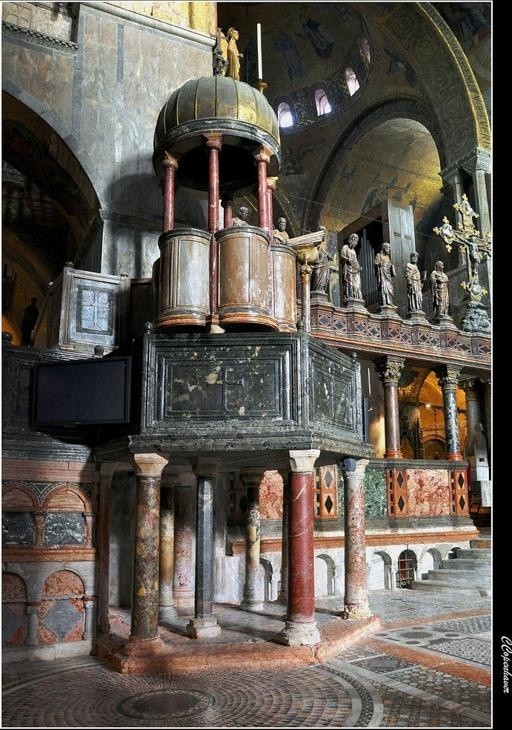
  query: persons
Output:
[229,204,251,227]
[338,231,368,312]
[310,224,336,305]
[404,250,430,323]
[214,25,229,76]
[294,14,334,59]
[272,215,290,248]
[427,258,458,329]
[372,241,402,318]
[224,25,244,80]
[21,297,40,346]
[466,421,488,459]
